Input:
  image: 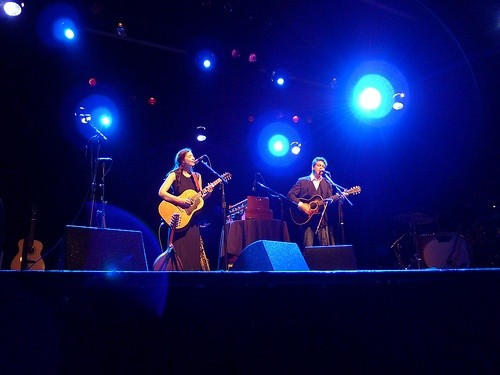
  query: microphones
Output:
[194,155,205,164]
[98,157,112,160]
[252,173,258,191]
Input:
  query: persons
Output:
[288,156,346,247]
[158,148,213,271]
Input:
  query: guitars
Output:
[152,215,184,272]
[9,201,46,270]
[156,171,231,232]
[288,185,361,225]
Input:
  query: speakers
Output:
[64,224,149,272]
[233,239,310,271]
[304,245,358,272]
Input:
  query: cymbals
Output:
[397,213,434,224]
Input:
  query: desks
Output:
[217,219,291,264]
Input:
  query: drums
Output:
[422,231,471,269]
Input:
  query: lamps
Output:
[391,92,407,111]
[196,126,207,141]
